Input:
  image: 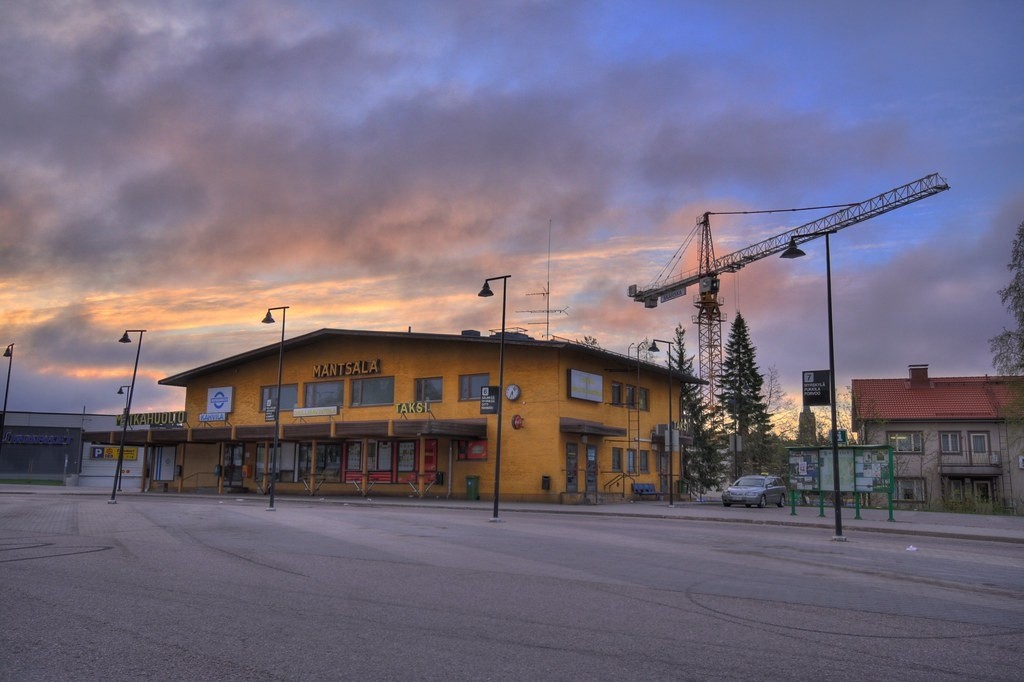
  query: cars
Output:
[722,473,788,509]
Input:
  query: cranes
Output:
[627,171,950,442]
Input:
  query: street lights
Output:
[1,341,15,450]
[477,273,513,520]
[779,230,847,542]
[77,405,93,473]
[115,384,133,492]
[106,329,147,504]
[648,338,674,508]
[260,305,293,511]
